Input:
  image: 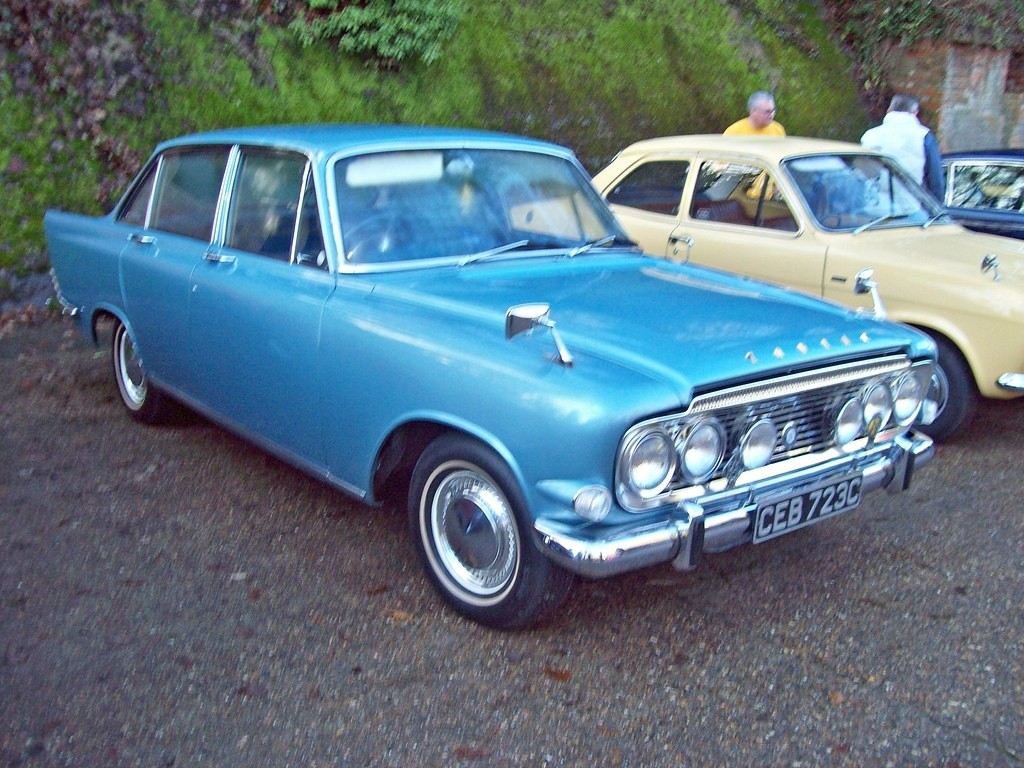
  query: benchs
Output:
[691,199,747,226]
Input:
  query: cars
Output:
[510,135,1024,447]
[811,148,1024,242]
[46,125,939,632]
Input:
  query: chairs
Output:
[271,208,318,255]
[760,215,798,233]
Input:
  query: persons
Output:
[721,92,786,201]
[859,93,946,217]
